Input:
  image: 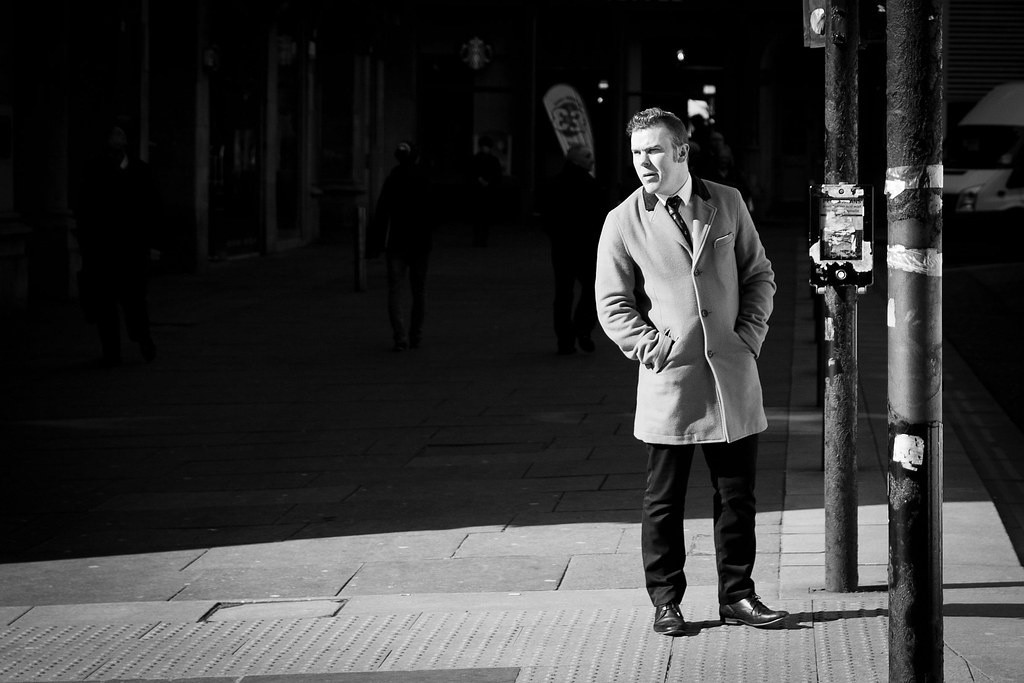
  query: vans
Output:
[940,81,1024,214]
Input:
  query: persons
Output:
[77,107,163,372]
[704,128,736,185]
[546,144,608,354]
[467,136,504,249]
[594,107,789,634]
[374,141,436,352]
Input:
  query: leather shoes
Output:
[654,602,686,636]
[719,593,791,628]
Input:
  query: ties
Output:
[664,196,692,249]
